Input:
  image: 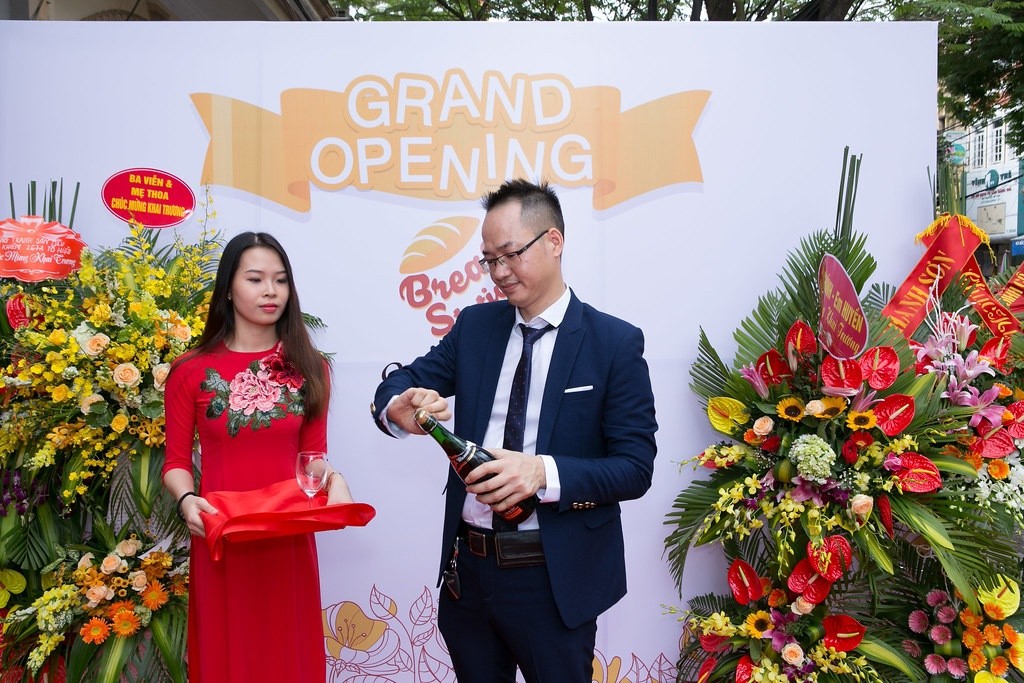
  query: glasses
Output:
[479,229,548,272]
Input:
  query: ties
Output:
[492,323,560,530]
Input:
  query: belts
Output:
[459,524,496,558]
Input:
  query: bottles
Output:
[415,408,540,526]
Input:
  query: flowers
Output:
[2,177,335,682]
[663,151,1023,683]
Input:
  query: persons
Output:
[369,177,658,683]
[161,232,353,683]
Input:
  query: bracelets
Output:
[326,472,346,491]
[176,491,196,522]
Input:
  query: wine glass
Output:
[295,451,329,510]
